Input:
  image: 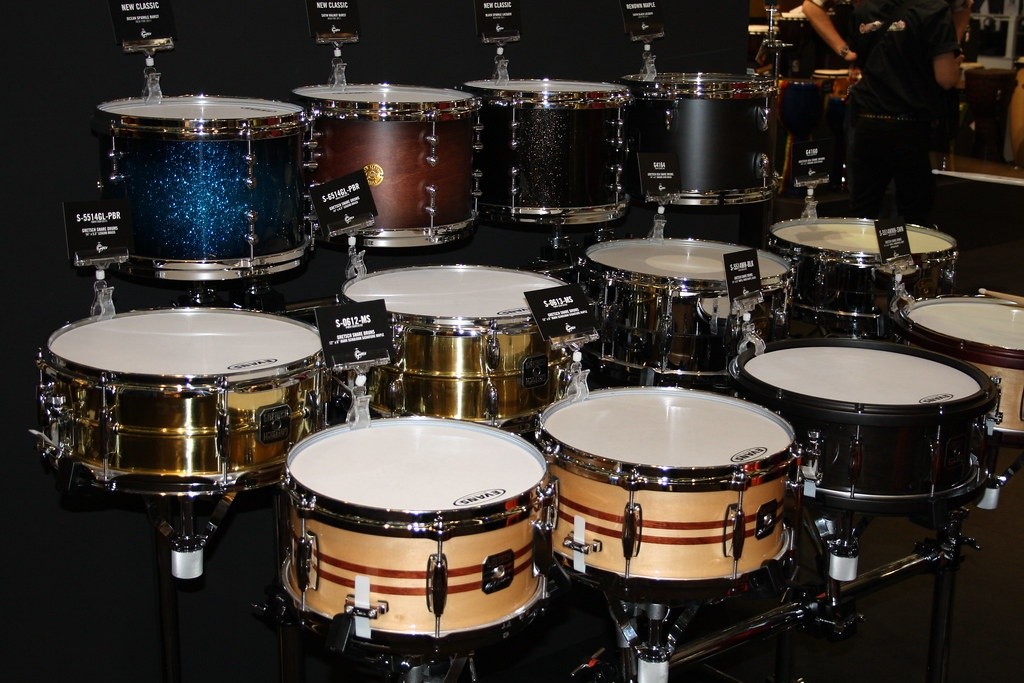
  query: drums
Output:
[732,339,997,507]
[623,71,792,207]
[90,95,313,282]
[577,238,791,377]
[281,416,551,637]
[38,305,326,499]
[454,78,637,227]
[535,384,798,580]
[299,80,490,249]
[337,265,574,424]
[767,214,961,338]
[890,290,1023,439]
